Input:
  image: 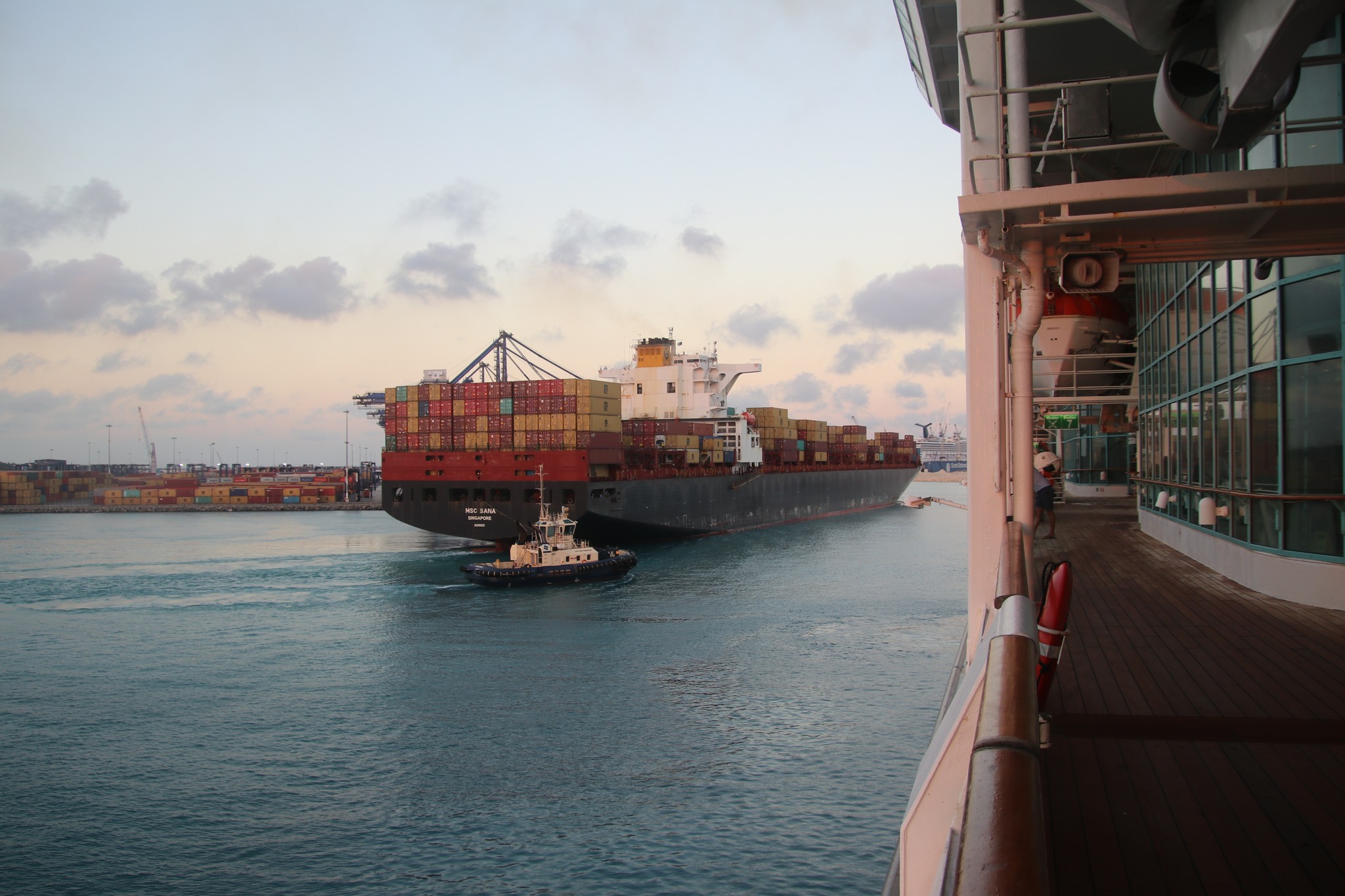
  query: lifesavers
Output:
[558,534,561,539]
[581,559,587,563]
[599,370,602,373]
[1039,471,1054,487]
[1035,561,1072,713]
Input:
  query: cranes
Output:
[137,406,162,473]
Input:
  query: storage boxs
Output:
[622,418,740,474]
[0,462,377,514]
[747,404,922,468]
[380,377,625,481]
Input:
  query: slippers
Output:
[1043,534,1056,539]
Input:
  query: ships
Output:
[351,328,926,548]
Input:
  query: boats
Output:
[459,462,638,592]
[913,423,967,473]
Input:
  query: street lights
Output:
[50,449,53,459]
[236,447,239,464]
[201,453,203,464]
[97,451,100,464]
[106,424,113,473]
[286,452,288,465]
[365,447,368,462]
[343,410,349,503]
[88,442,91,471]
[209,444,212,469]
[256,449,259,467]
[178,452,181,472]
[211,443,215,467]
[359,447,361,467]
[172,437,178,474]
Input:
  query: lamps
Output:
[1098,469,1106,481]
[1152,488,1177,512]
[1065,472,1074,480]
[1195,495,1229,531]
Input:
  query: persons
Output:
[1034,466,1056,539]
